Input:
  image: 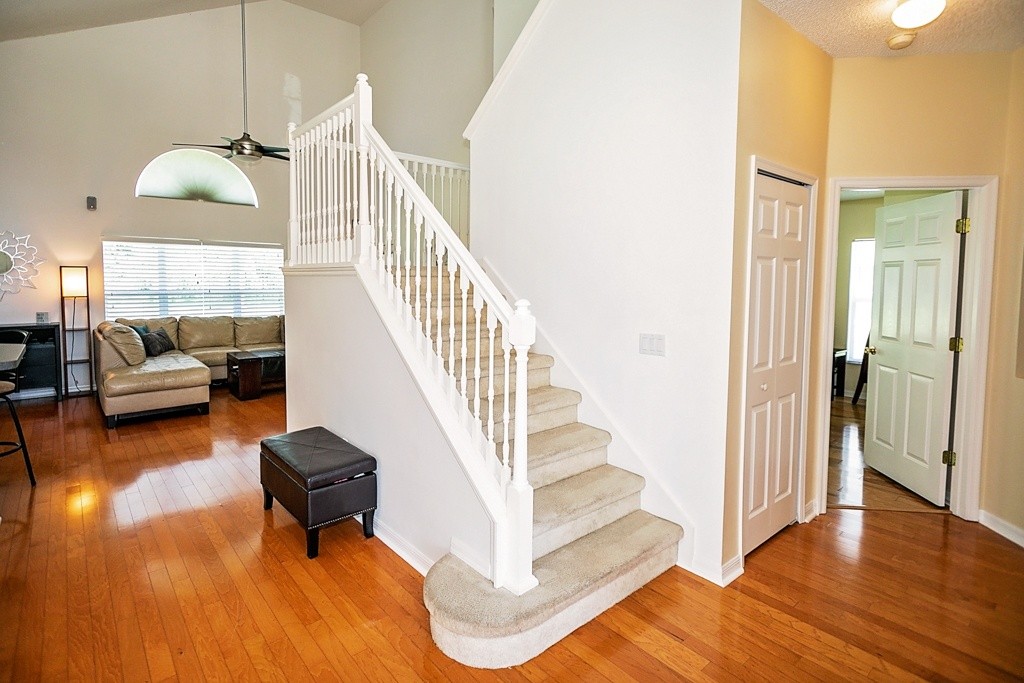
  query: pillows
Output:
[129,324,150,337]
[142,326,177,355]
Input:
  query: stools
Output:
[0,380,38,489]
[259,425,379,560]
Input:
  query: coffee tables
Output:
[226,350,285,401]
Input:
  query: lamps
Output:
[890,0,946,30]
[60,264,95,402]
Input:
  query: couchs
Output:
[92,315,286,430]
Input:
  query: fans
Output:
[172,0,301,163]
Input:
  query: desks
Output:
[831,348,847,400]
[0,342,28,371]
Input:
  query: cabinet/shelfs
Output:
[0,321,64,401]
